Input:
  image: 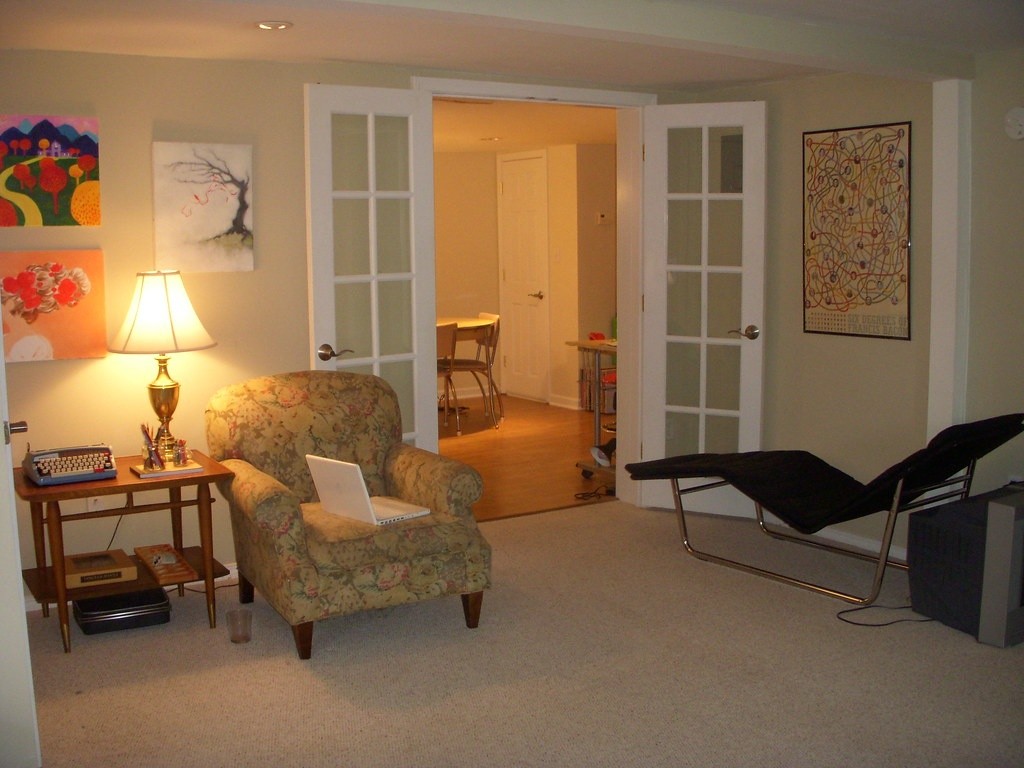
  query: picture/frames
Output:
[801,118,911,337]
[148,139,256,277]
[0,249,109,363]
[0,113,103,229]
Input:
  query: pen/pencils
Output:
[140,421,166,471]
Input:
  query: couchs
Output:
[205,370,491,662]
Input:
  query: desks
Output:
[13,445,230,657]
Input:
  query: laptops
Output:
[306,454,432,527]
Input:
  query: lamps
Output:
[104,269,218,447]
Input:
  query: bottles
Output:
[142,440,168,472]
[173,440,189,468]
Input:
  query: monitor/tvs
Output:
[906,478,1024,648]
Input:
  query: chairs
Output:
[622,411,1022,609]
[435,312,504,439]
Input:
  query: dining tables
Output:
[566,337,616,465]
